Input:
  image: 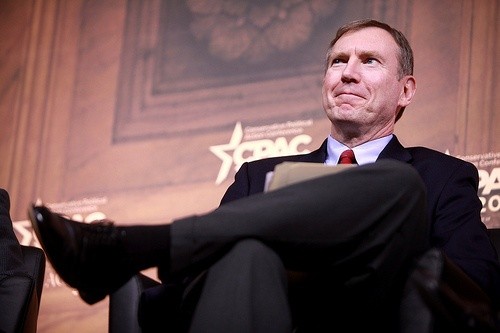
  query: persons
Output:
[27,18,494,333]
[1,186,38,332]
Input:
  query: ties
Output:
[338,150,354,164]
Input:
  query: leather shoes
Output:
[28,203,122,305]
